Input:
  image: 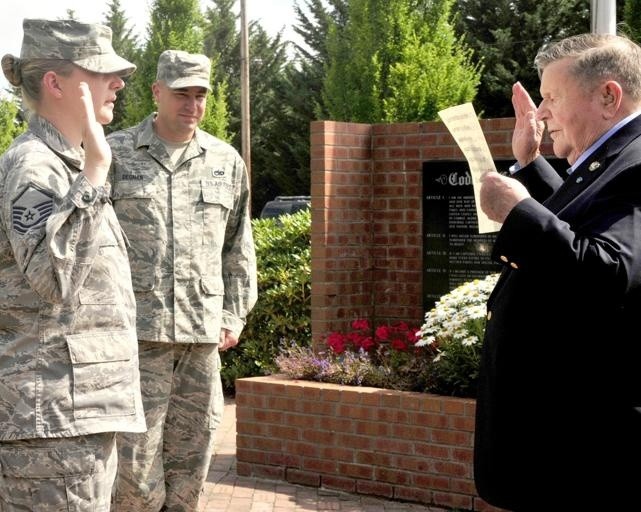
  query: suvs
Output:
[260,193,313,219]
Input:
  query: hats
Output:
[20,17,138,77]
[157,50,213,93]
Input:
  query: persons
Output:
[472,31,640,512]
[101,49,260,511]
[1,20,148,512]
